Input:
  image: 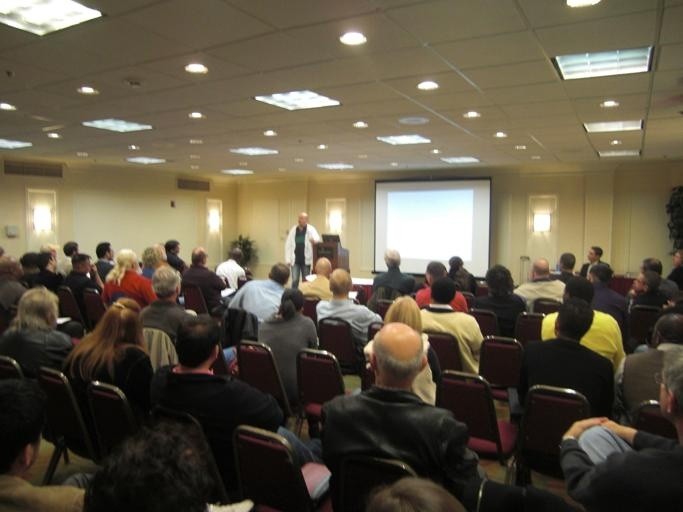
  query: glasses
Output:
[652,371,666,386]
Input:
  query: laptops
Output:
[323,236,341,247]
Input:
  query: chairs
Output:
[0,267,682,511]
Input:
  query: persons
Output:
[285,212,323,288]
